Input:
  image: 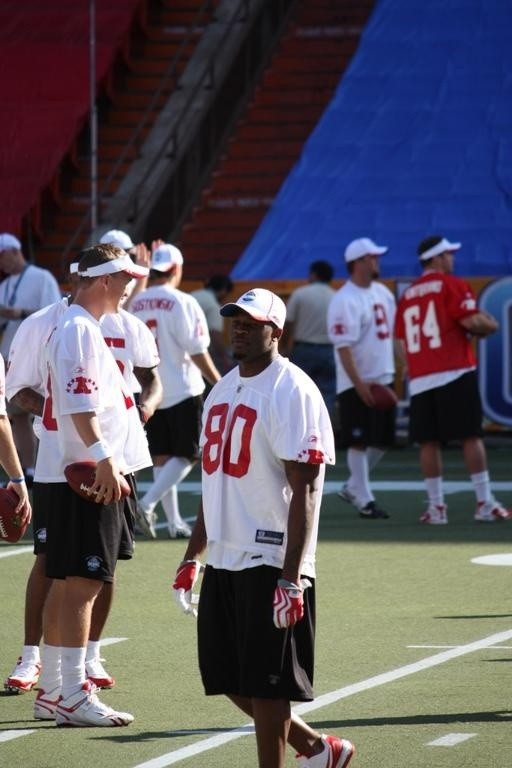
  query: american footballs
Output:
[0,487,27,543]
[65,461,131,503]
[371,385,398,408]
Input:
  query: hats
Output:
[344,237,388,263]
[0,232,22,253]
[76,253,151,279]
[148,243,184,273]
[219,288,287,331]
[98,229,135,252]
[69,261,79,275]
[417,236,462,263]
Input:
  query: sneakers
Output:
[359,500,389,519]
[54,678,135,728]
[167,523,193,539]
[135,502,158,539]
[417,502,448,526]
[33,686,61,720]
[473,497,512,523]
[336,484,355,503]
[3,655,43,693]
[84,656,116,689]
[294,733,355,768]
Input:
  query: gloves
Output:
[172,558,206,619]
[271,577,313,630]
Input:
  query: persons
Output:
[324,234,398,519]
[393,237,509,523]
[175,288,355,767]
[0,229,234,538]
[5,253,164,695]
[36,245,150,725]
[275,259,337,423]
